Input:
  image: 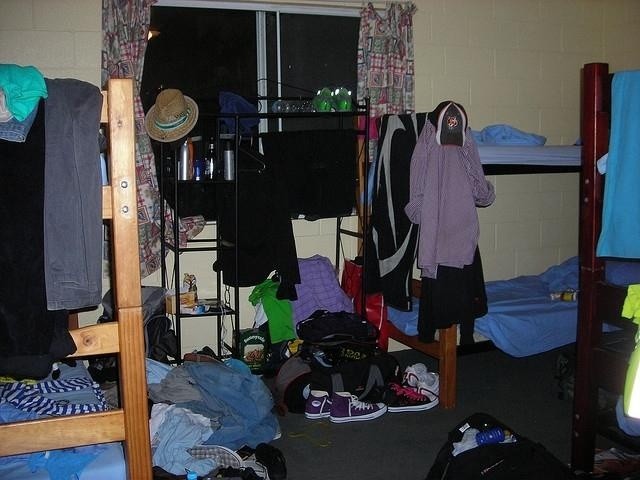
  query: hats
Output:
[145,88,199,143]
[430,101,467,148]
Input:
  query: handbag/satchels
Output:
[88,286,178,382]
[427,415,577,479]
[297,310,379,371]
[343,258,389,353]
[272,345,402,416]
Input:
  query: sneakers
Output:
[395,362,439,393]
[313,87,352,112]
[306,389,332,420]
[385,383,439,413]
[187,443,287,480]
[330,392,386,423]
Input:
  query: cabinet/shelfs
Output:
[158,77,373,378]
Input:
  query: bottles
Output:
[179,135,236,181]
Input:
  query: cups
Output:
[272,95,353,113]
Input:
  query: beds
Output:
[0,75,154,479]
[355,101,640,472]
